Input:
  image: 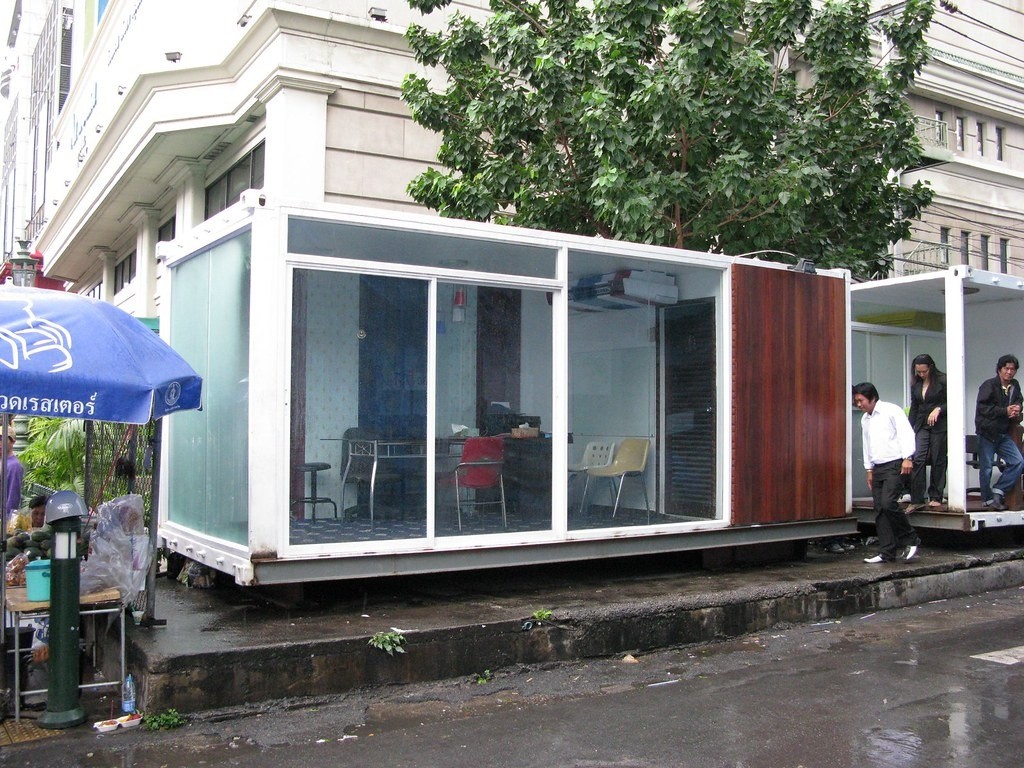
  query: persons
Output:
[826,354,946,563]
[974,355,1024,509]
[0,426,47,531]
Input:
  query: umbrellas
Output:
[0,287,202,642]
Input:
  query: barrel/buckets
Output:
[24,559,51,602]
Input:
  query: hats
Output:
[0,425,16,442]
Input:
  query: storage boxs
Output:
[25,560,50,601]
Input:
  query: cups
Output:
[132,611,144,625]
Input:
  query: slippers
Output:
[929,499,941,507]
[905,502,925,514]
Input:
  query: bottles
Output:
[121,676,136,713]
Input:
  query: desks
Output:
[5,584,126,731]
[320,433,575,533]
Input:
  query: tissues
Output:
[451,424,479,437]
[511,422,539,439]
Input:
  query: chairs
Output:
[436,437,507,532]
[578,438,651,519]
[340,427,403,522]
[966,435,1003,496]
[568,440,620,514]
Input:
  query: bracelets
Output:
[866,470,874,472]
[937,406,941,412]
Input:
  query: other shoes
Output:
[863,556,887,563]
[982,493,1009,510]
[907,537,921,559]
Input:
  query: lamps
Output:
[368,7,387,19]
[240,15,250,27]
[45,491,89,524]
[739,250,818,275]
[165,52,182,61]
[451,284,467,325]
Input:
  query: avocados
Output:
[3,530,54,560]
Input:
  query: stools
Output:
[291,462,338,523]
